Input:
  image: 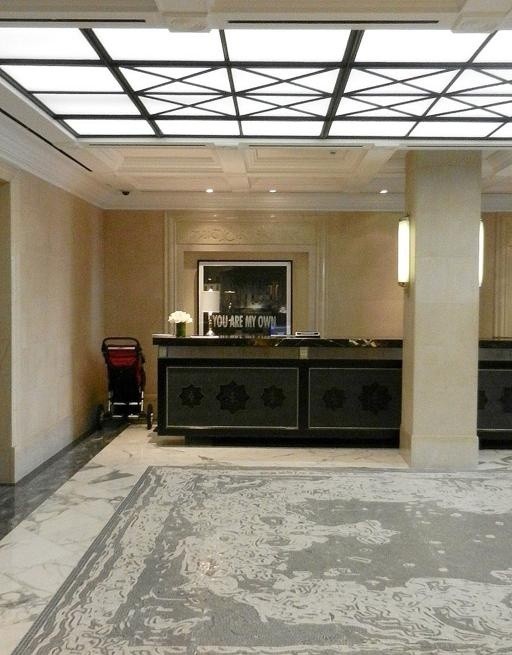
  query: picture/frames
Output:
[196,259,294,337]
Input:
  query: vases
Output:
[176,321,187,337]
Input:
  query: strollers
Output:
[93,337,153,431]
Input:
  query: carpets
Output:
[11,462,512,653]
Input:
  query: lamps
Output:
[396,211,410,289]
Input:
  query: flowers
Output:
[168,310,193,325]
